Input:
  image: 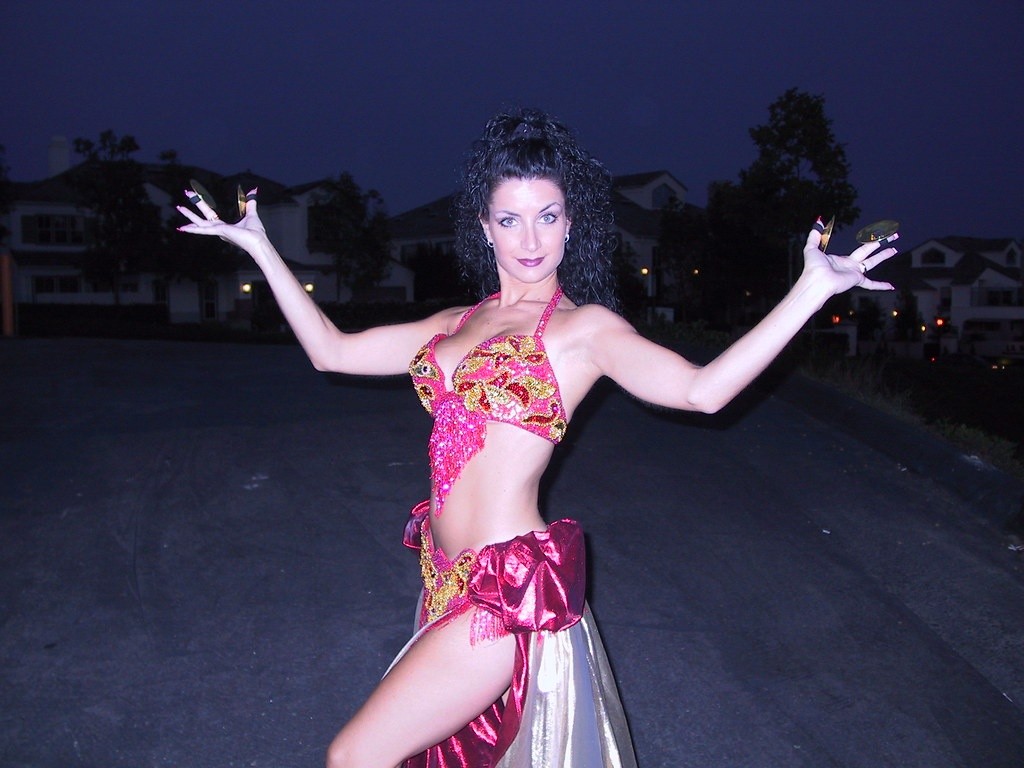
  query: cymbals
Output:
[812,211,903,256]
[183,176,260,217]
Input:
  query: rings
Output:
[208,215,219,222]
[860,263,867,274]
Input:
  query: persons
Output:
[176,106,898,768]
